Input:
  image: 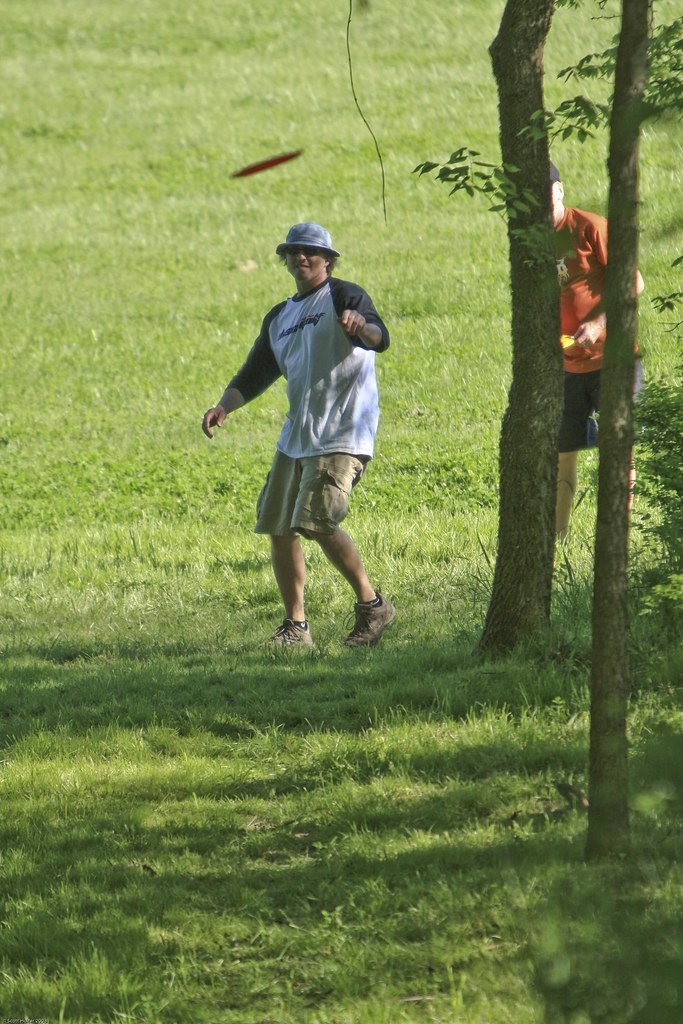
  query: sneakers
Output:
[257,619,314,649]
[345,591,397,647]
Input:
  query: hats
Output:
[549,161,559,181]
[276,223,341,258]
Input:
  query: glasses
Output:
[285,247,324,256]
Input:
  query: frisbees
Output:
[560,335,574,349]
[232,151,300,177]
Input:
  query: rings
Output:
[582,341,587,346]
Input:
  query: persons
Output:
[200,224,393,650]
[551,159,643,548]
